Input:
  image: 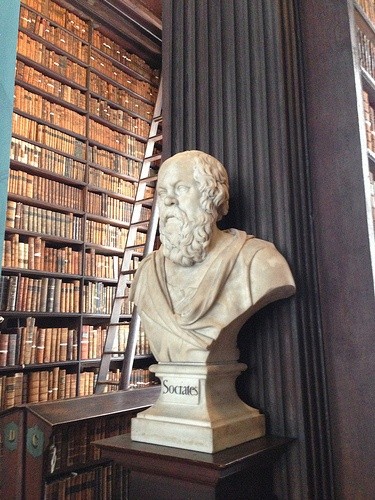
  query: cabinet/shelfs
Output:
[297,0,375,246]
[1,0,164,412]
[22,386,161,500]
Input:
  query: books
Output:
[43,416,141,499]
[353,0,375,244]
[0,0,163,411]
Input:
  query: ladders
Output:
[89,66,162,397]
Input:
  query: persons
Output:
[128,150,295,365]
